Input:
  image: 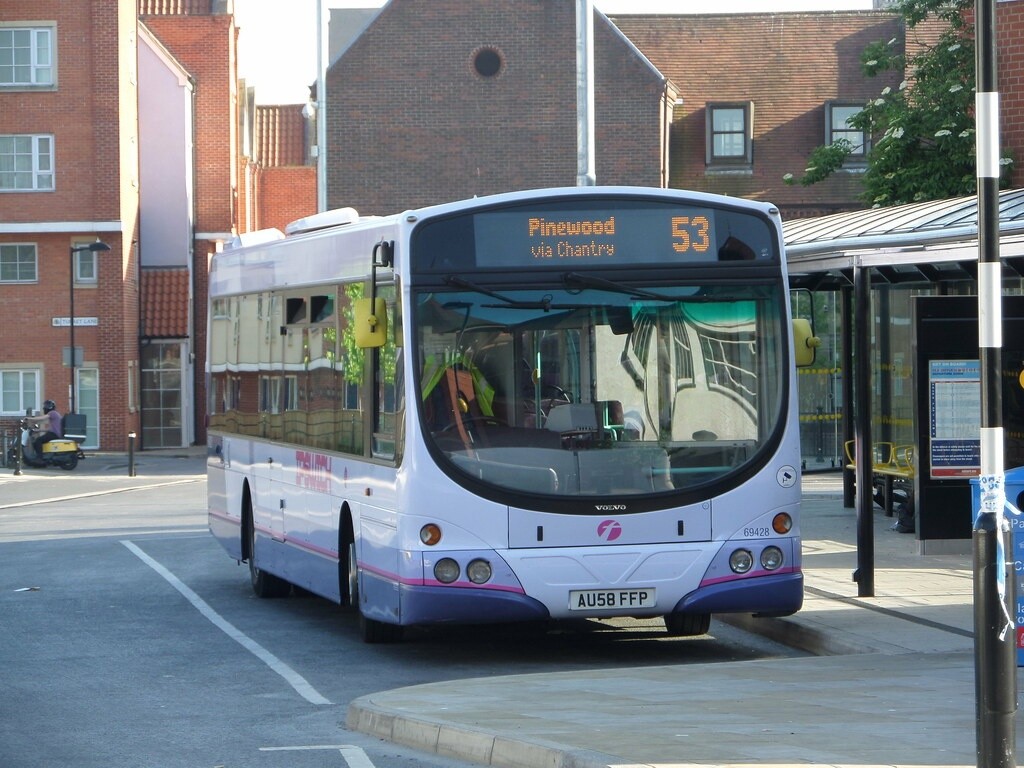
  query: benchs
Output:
[845,440,914,517]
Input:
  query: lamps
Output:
[128,431,137,438]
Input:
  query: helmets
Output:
[43,400,55,414]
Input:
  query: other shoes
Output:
[36,454,43,464]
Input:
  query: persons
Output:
[23,400,61,457]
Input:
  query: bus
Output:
[202,181,821,635]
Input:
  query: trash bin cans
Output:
[969,466,1024,667]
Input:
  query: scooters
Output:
[5,420,86,471]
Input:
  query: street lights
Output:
[68,241,111,460]
